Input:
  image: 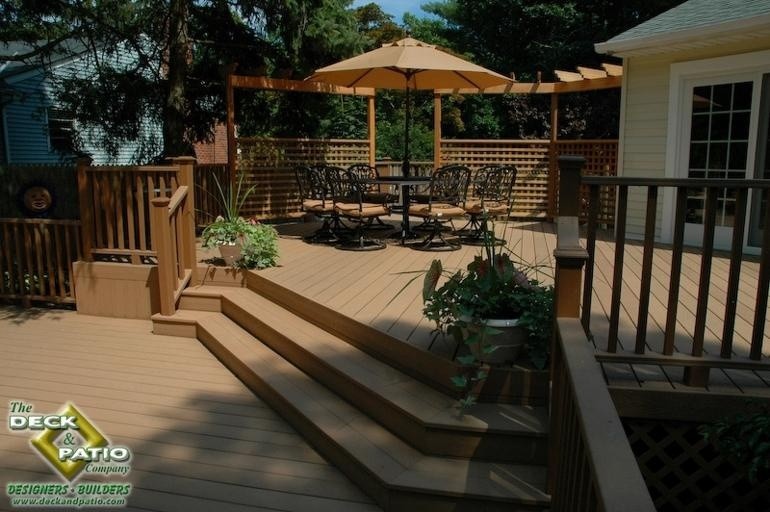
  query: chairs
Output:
[293,163,515,252]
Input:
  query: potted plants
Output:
[197,170,278,269]
[424,210,556,408]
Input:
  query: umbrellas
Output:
[304,34,520,231]
[691,95,721,110]
